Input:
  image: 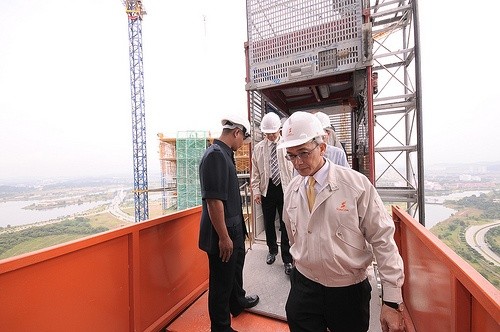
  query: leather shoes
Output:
[266,251,277,264]
[234,295,259,317]
[284,262,293,275]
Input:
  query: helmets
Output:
[221,113,250,134]
[260,112,281,133]
[314,112,331,129]
[277,111,326,149]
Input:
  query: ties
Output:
[307,176,317,213]
[271,137,280,186]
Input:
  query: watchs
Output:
[383,301,405,312]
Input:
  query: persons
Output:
[278,111,405,332]
[250,112,300,275]
[199,115,259,332]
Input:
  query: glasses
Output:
[285,143,320,161]
